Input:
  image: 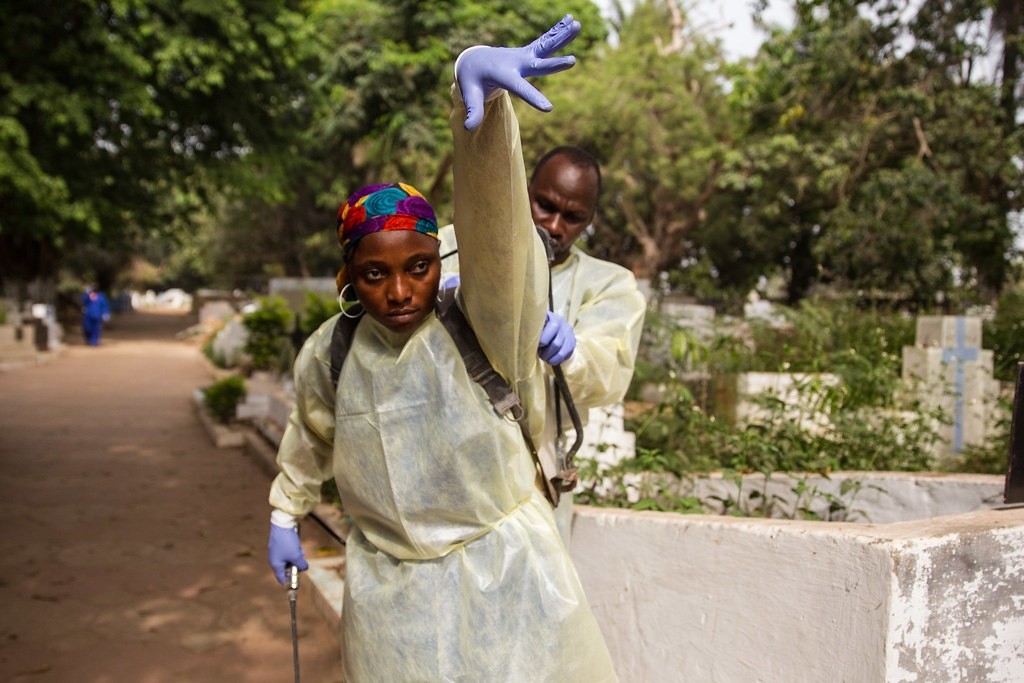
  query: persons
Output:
[80,286,112,346]
[434,145,647,432]
[269,12,619,683]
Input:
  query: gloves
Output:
[268,523,309,586]
[455,13,582,131]
[537,310,576,365]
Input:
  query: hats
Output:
[337,182,439,261]
